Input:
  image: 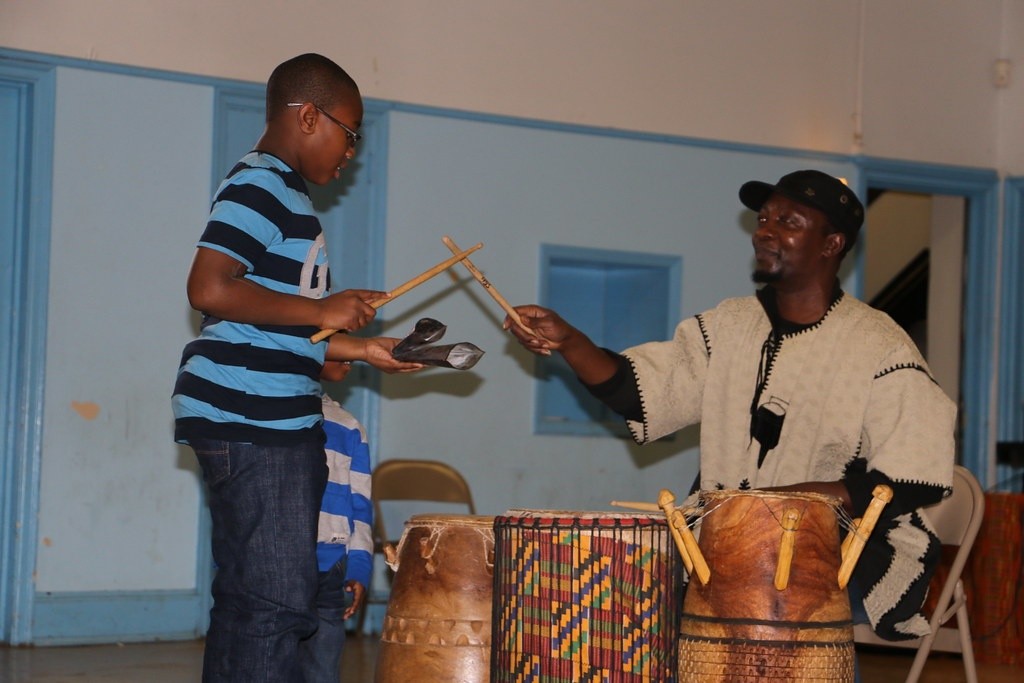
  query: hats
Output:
[737,169,865,252]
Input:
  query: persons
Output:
[170,54,428,683]
[300,359,373,683]
[505,172,960,682]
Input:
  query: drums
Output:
[376,514,501,683]
[677,491,856,681]
[490,508,679,682]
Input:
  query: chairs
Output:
[353,459,478,639]
[851,463,985,682]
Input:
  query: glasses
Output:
[287,101,362,149]
